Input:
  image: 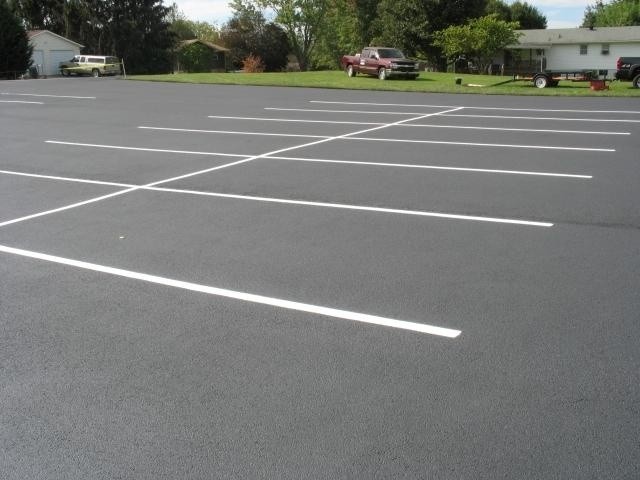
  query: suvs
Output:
[58,55,121,78]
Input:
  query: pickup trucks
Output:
[342,46,420,81]
[616,55,640,88]
